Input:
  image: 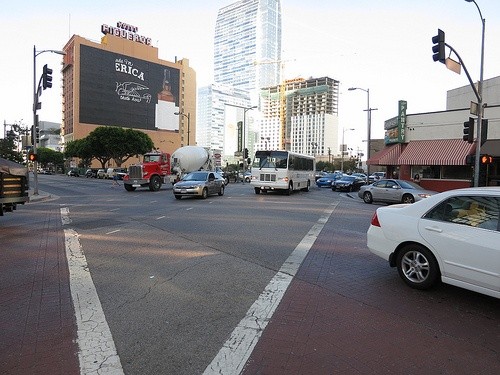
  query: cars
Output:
[212,164,251,185]
[28,166,127,180]
[316,174,336,188]
[173,171,226,200]
[358,179,440,206]
[366,187,500,298]
[314,169,386,186]
[332,175,366,193]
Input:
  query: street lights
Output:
[464,0,485,189]
[33,43,68,197]
[173,112,190,147]
[348,86,378,188]
[243,105,258,183]
[342,128,355,173]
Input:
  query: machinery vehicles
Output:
[122,146,212,192]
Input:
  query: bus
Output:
[247,150,316,195]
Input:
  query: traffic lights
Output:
[462,117,475,144]
[43,64,54,90]
[480,155,492,164]
[28,154,36,161]
[431,29,447,64]
[31,125,40,145]
